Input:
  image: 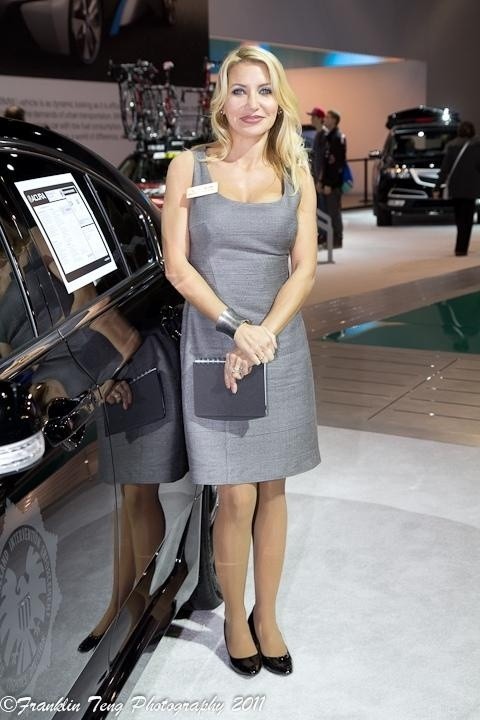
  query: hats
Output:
[306,108,325,118]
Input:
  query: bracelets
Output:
[215,306,252,341]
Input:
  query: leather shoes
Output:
[76,633,101,651]
[223,619,263,677]
[248,607,292,676]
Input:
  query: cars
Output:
[115,146,193,219]
[1,115,223,719]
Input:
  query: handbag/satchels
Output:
[433,183,449,202]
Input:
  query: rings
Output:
[232,367,244,373]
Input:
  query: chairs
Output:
[397,136,416,152]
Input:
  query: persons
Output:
[438,299,470,353]
[0,218,190,654]
[305,106,330,244]
[431,121,477,256]
[316,109,348,250]
[159,40,321,679]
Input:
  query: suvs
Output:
[368,105,479,225]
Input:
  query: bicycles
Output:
[108,57,217,144]
